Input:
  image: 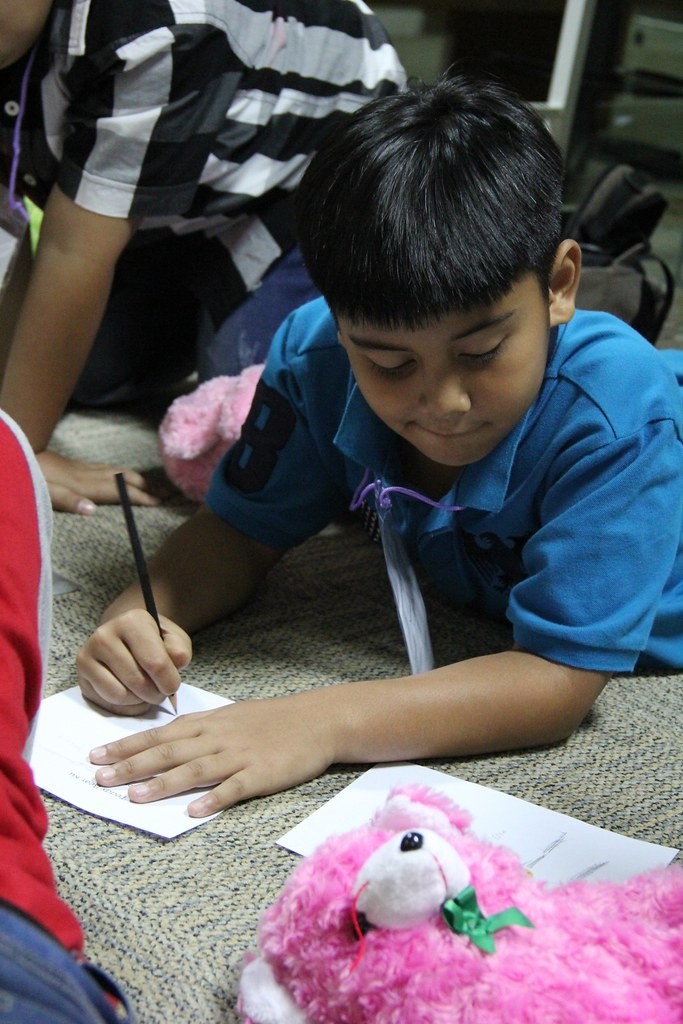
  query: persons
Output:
[0,0,411,517]
[0,408,141,1024]
[73,66,683,818]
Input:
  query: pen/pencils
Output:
[114,472,181,717]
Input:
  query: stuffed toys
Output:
[235,782,683,1024]
[158,363,266,503]
